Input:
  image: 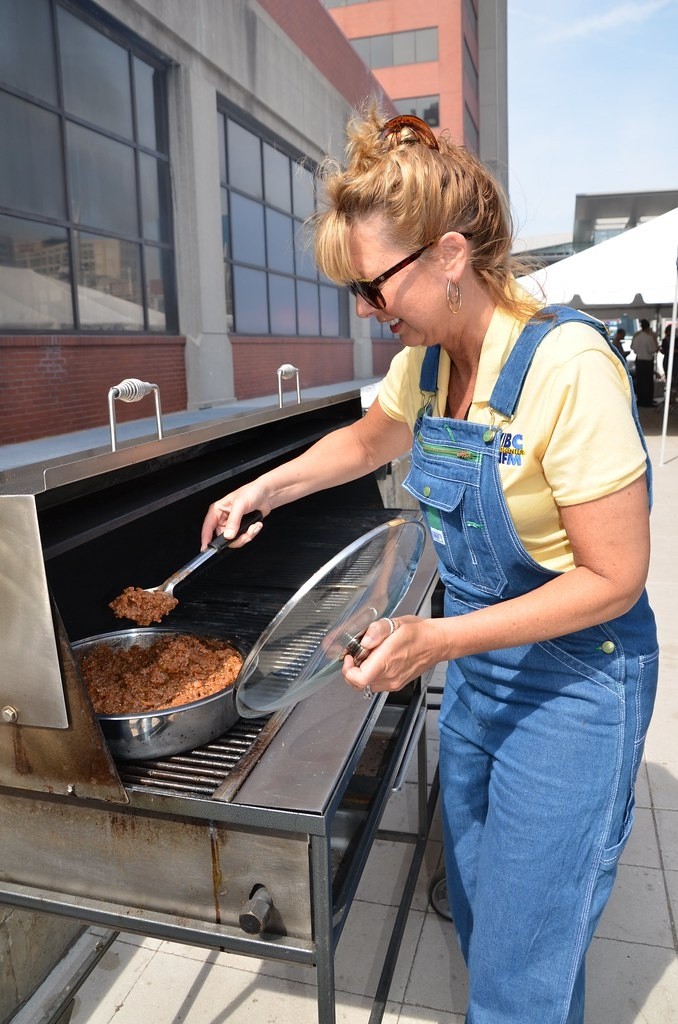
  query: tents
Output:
[515,208,678,464]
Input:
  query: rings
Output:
[384,618,395,633]
[369,607,377,615]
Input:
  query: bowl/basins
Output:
[70,625,259,760]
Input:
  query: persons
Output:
[630,319,658,408]
[321,526,403,659]
[660,325,678,384]
[200,115,660,1024]
[612,329,630,359]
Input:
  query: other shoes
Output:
[646,403,659,408]
[432,877,453,921]
[652,400,657,404]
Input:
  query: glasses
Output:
[346,232,473,310]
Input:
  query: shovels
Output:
[108,509,263,626]
